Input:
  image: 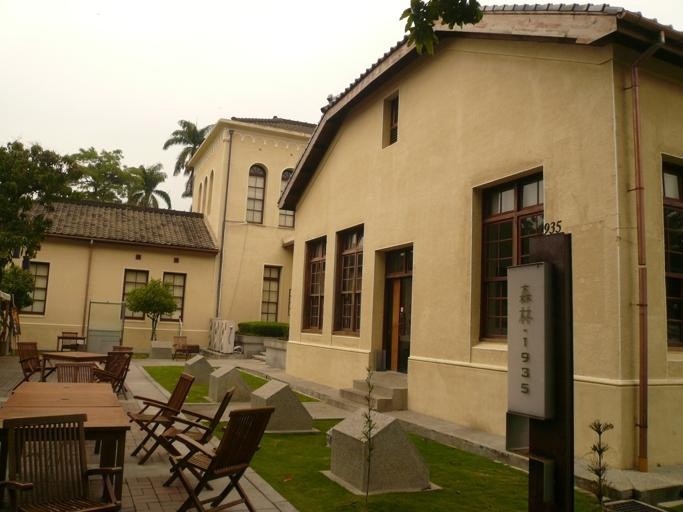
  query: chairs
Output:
[2,413,88,511]
[172,335,191,361]
[161,406,275,512]
[126,372,196,432]
[10,330,134,401]
[130,385,238,466]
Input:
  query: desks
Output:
[0,382,131,511]
